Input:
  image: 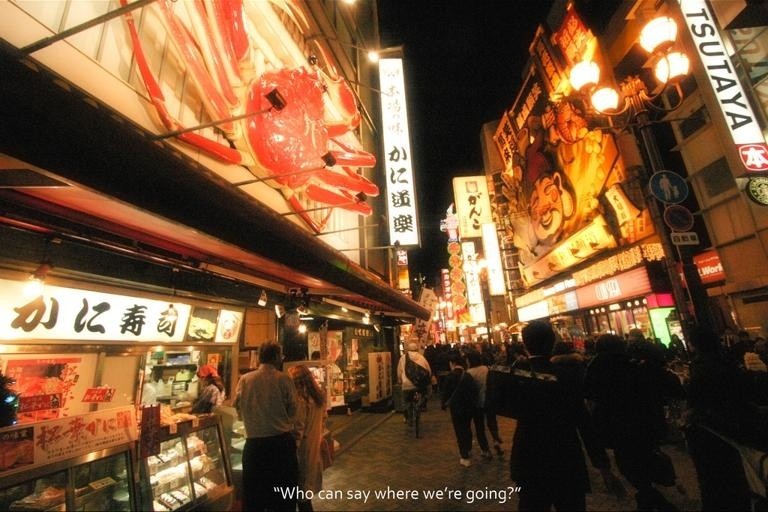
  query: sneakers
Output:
[481,450,492,458]
[494,441,504,456]
[460,458,473,467]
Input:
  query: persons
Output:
[286,366,329,512]
[191,366,223,415]
[232,341,301,511]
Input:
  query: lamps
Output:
[372,322,382,332]
[295,299,311,316]
[255,289,270,308]
[272,303,288,319]
[27,259,58,284]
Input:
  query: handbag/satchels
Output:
[321,432,335,468]
[405,353,430,389]
[484,355,558,428]
[740,446,768,511]
[649,447,675,488]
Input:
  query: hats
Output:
[407,343,418,350]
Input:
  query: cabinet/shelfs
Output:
[0,438,143,511]
[142,410,238,510]
[150,362,196,402]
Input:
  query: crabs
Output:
[121,0,380,235]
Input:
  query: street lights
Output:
[462,256,493,347]
[567,13,726,339]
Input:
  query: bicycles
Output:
[408,389,427,438]
